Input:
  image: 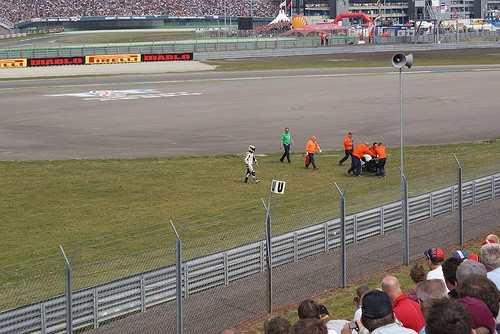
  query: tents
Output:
[281,25,347,36]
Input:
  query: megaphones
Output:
[391,52,407,69]
[405,54,413,69]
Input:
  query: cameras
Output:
[348,322,358,329]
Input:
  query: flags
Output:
[286,2,291,10]
[276,6,280,13]
[280,0,286,8]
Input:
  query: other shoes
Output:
[254,179,260,184]
[244,176,248,183]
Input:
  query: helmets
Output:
[249,146,255,152]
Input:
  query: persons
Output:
[346,141,375,176]
[243,145,260,184]
[321,33,328,47]
[369,143,377,159]
[305,136,321,169]
[218,234,500,334]
[339,132,354,166]
[0,0,293,38]
[373,143,386,178]
[280,127,294,163]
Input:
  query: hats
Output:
[457,297,496,334]
[424,247,445,263]
[482,239,494,246]
[361,290,392,318]
[453,250,468,259]
[318,304,329,317]
